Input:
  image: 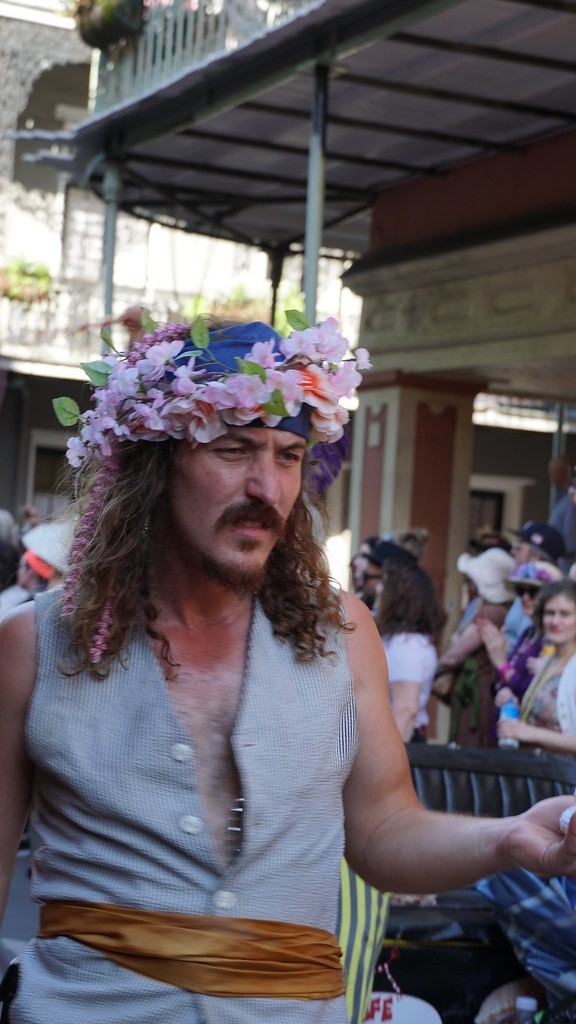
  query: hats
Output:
[507,519,566,560]
[23,550,56,580]
[456,547,516,603]
[363,539,419,567]
[504,561,565,587]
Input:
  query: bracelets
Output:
[497,664,512,675]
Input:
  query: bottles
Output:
[511,996,537,1024]
[498,698,519,750]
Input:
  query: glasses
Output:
[362,572,383,584]
[514,585,539,599]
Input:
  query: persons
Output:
[0,317,576,1024]
[432,453,576,755]
[350,527,448,746]
[0,502,82,619]
